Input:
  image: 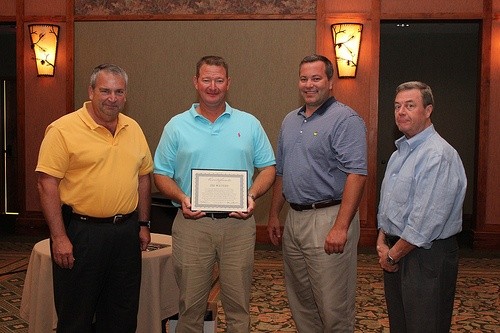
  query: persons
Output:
[268,53,368,333]
[152,56,277,333]
[377,81,468,333]
[35,65,154,333]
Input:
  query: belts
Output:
[68,213,136,224]
[289,199,342,211]
[201,213,231,219]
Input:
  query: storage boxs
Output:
[166,301,218,333]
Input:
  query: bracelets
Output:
[248,194,255,201]
[138,220,150,228]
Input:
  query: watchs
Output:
[387,250,397,264]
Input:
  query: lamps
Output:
[27,23,61,77]
[330,22,365,79]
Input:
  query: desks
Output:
[20,232,221,333]
